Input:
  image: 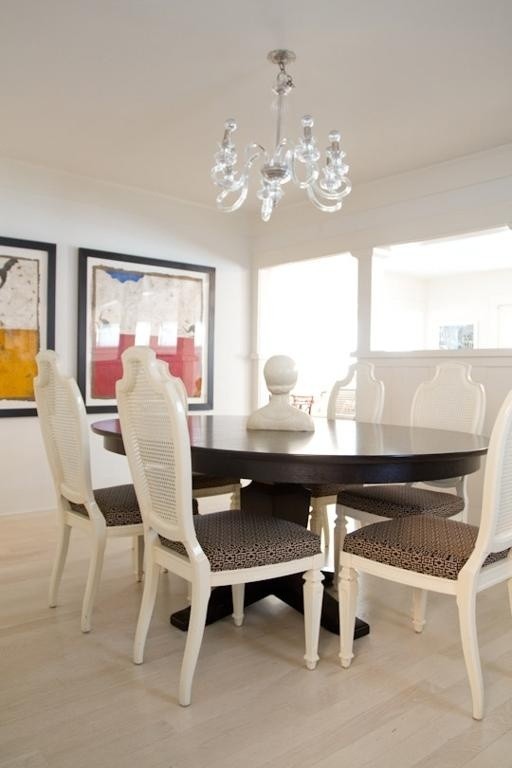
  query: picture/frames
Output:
[0,240,216,420]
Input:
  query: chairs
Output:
[331,361,487,632]
[129,365,237,511]
[338,391,511,721]
[305,361,385,555]
[115,346,327,706]
[31,350,203,632]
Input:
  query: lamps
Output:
[211,50,352,222]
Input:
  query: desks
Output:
[90,418,483,642]
